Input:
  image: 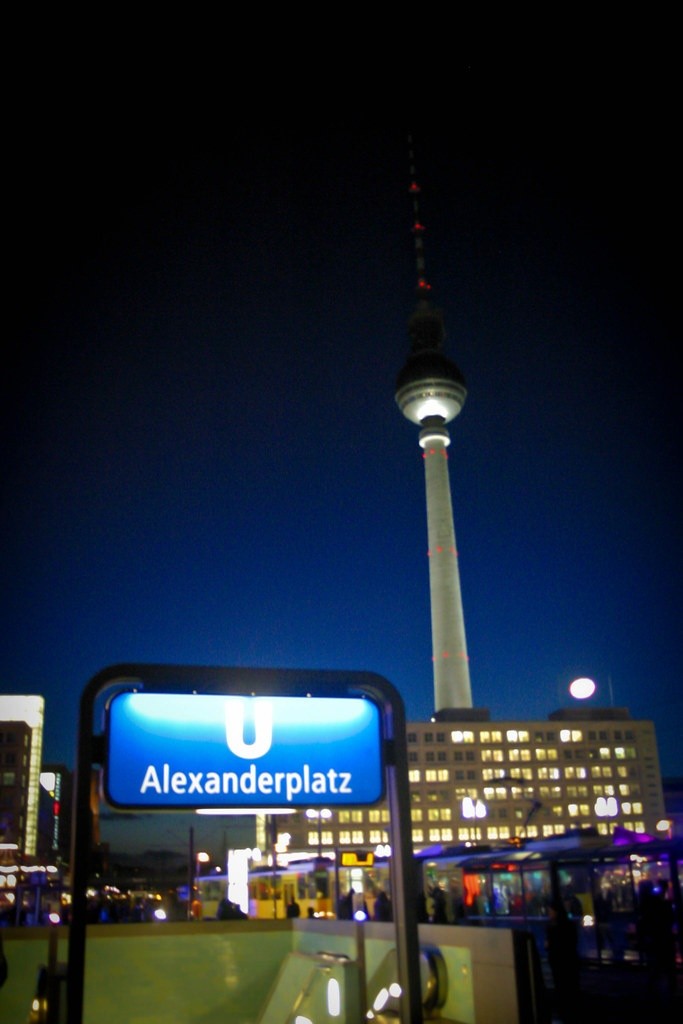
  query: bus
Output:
[412,825,683,999]
[195,856,390,920]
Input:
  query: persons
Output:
[638,878,677,990]
[374,891,392,920]
[343,889,354,919]
[217,900,248,919]
[308,907,316,918]
[9,895,186,924]
[417,887,614,1024]
[287,896,300,918]
